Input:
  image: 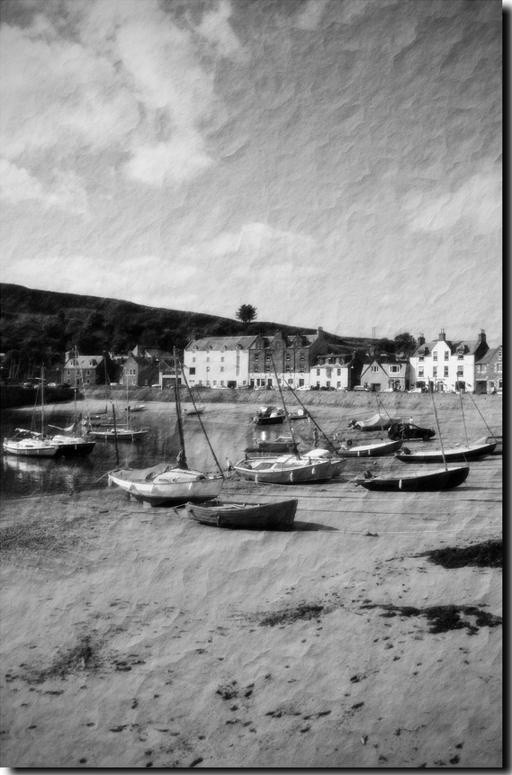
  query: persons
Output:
[244,461,252,470]
[399,422,410,442]
[409,416,415,428]
[225,456,235,479]
[313,427,319,448]
[339,436,350,451]
[251,411,260,424]
[362,467,374,480]
